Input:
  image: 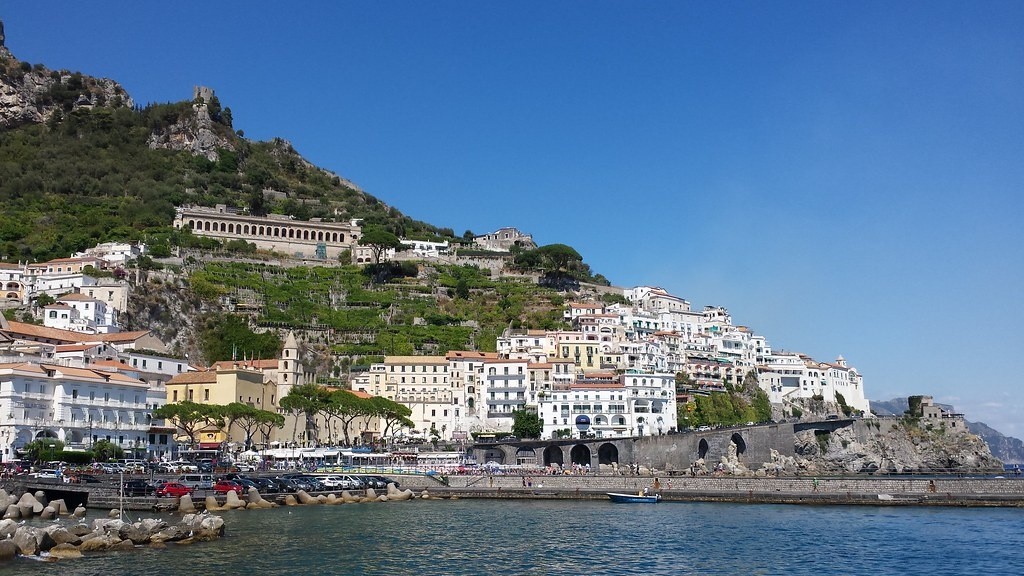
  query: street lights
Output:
[49,445,56,469]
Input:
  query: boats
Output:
[606,492,662,503]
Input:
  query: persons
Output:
[244,458,319,471]
[93,460,98,475]
[811,477,820,492]
[54,468,61,482]
[449,462,723,497]
[1013,463,1020,477]
[928,480,936,493]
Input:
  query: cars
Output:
[301,477,326,491]
[29,469,63,478]
[316,475,400,490]
[745,421,753,426]
[248,478,278,493]
[232,462,256,472]
[289,478,312,492]
[150,479,169,487]
[92,459,215,474]
[66,474,102,483]
[157,483,199,495]
[700,425,711,432]
[271,478,299,492]
[213,480,244,495]
[232,479,264,493]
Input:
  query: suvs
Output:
[116,481,157,497]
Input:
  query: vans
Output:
[177,475,219,489]
